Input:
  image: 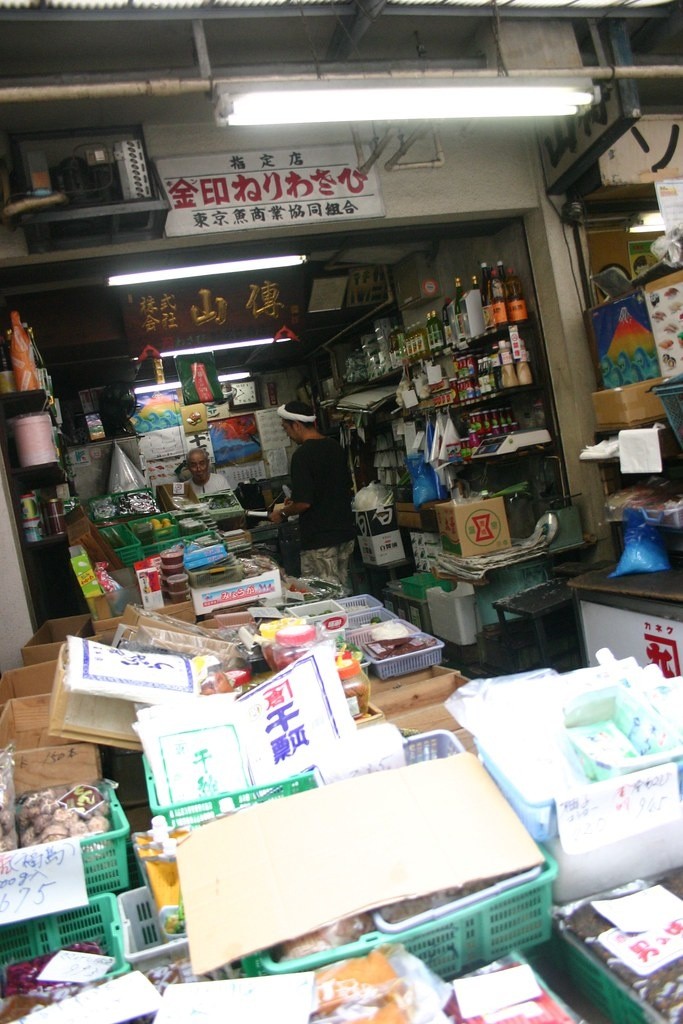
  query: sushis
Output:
[649,287,683,370]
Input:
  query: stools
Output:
[491,575,575,668]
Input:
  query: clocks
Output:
[226,378,263,410]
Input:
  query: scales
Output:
[534,492,584,550]
[471,428,552,458]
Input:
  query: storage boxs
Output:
[21,613,93,665]
[127,511,181,546]
[89,488,159,523]
[425,580,484,645]
[156,482,201,513]
[401,573,452,599]
[197,489,244,521]
[66,517,124,572]
[82,567,141,621]
[187,568,283,616]
[93,589,196,636]
[97,524,145,573]
[0,647,683,1024]
[69,545,114,620]
[592,259,683,528]
[141,530,228,560]
[435,496,513,558]
[136,560,164,609]
[351,505,406,565]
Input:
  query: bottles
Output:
[0,311,51,396]
[388,262,532,460]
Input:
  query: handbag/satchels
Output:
[405,454,448,510]
[608,508,670,578]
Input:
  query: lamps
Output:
[160,335,296,358]
[105,253,309,287]
[134,371,254,395]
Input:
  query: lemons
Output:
[151,518,173,530]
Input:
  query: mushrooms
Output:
[0,785,116,853]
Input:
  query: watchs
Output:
[280,508,288,522]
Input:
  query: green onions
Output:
[385,470,411,504]
[490,480,531,498]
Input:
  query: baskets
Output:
[0,892,132,1000]
[117,887,190,971]
[98,512,227,567]
[284,594,445,680]
[141,754,319,828]
[549,935,666,1024]
[242,730,559,985]
[0,783,132,897]
[652,374,683,450]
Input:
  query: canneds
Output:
[17,492,68,543]
[428,375,461,408]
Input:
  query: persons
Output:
[268,401,357,601]
[183,448,230,498]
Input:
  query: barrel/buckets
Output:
[10,411,59,468]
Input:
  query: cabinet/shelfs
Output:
[0,388,69,547]
[337,309,571,504]
